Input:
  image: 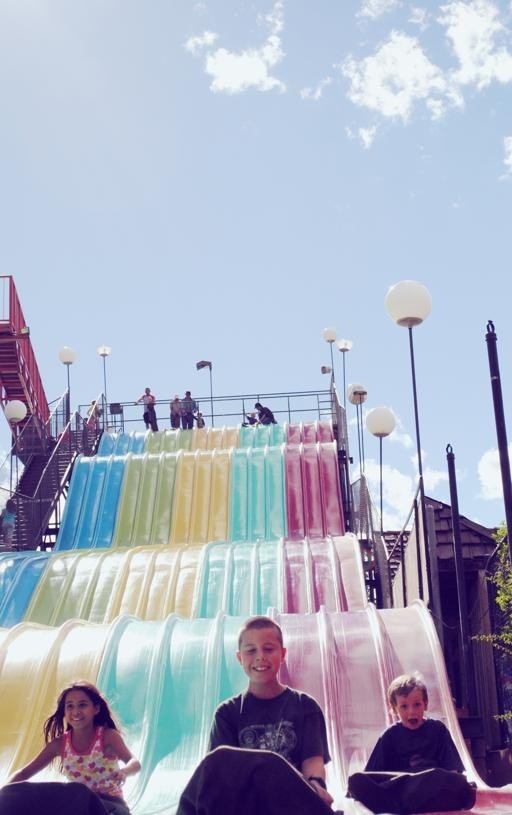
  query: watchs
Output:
[307,776,327,790]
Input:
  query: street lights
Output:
[197,361,213,427]
[321,328,397,536]
[4,400,28,552]
[387,280,434,607]
[59,348,74,484]
[98,345,111,430]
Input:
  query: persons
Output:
[193,412,205,428]
[7,681,142,799]
[87,400,101,430]
[254,403,277,425]
[181,391,197,429]
[135,388,155,430]
[245,412,258,424]
[207,617,334,806]
[364,676,465,775]
[170,394,183,429]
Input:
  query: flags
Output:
[197,361,211,370]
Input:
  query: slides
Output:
[0,420,512,815]
